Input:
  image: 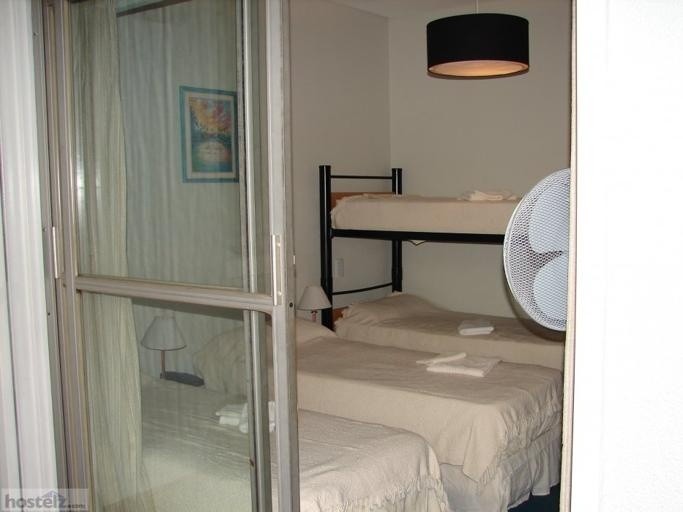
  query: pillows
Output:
[210,318,336,358]
[342,292,435,322]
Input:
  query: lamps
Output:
[425,0,529,80]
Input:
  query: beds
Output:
[137,369,450,510]
[318,167,565,369]
[191,317,564,509]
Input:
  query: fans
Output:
[504,170,569,331]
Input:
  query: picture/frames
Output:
[178,83,240,184]
